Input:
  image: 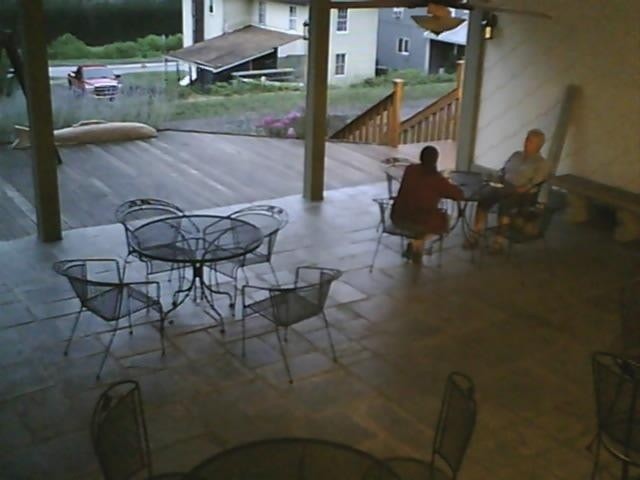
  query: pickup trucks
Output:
[67,63,124,103]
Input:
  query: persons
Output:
[461,126,551,255]
[391,142,463,265]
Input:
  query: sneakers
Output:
[401,241,423,267]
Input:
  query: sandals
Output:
[462,234,479,251]
[487,240,505,256]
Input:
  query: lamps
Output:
[478,10,499,41]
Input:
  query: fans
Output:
[330,1,554,38]
